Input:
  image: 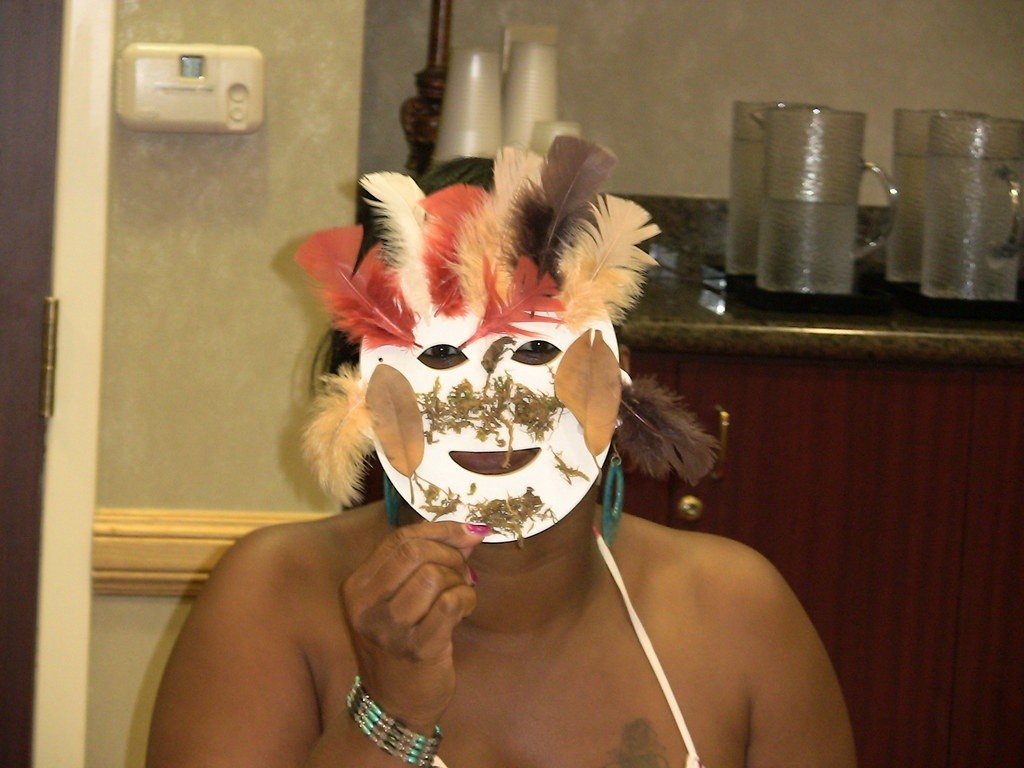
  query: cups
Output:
[436,39,582,163]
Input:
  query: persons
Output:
[146,159,858,768]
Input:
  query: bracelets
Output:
[345,676,440,768]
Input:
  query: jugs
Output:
[751,103,896,295]
[919,110,1024,299]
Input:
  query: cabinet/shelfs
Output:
[605,350,1024,768]
